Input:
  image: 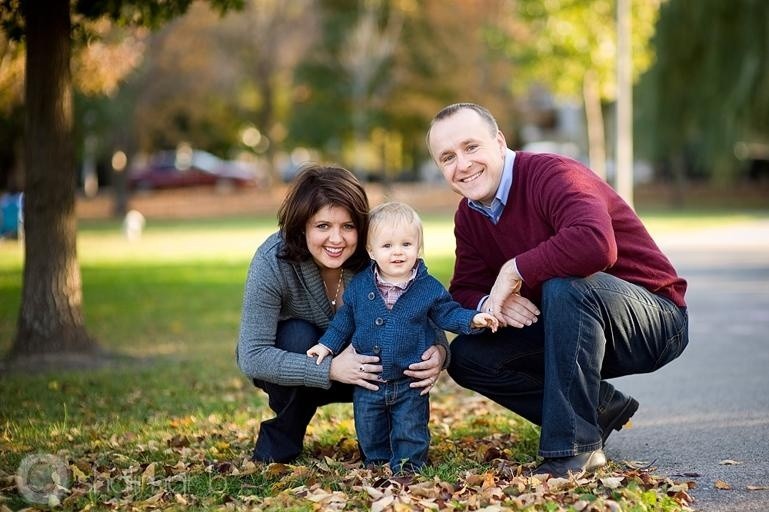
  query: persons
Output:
[422,100,687,477]
[303,203,498,483]
[237,165,454,465]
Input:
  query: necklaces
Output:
[319,264,344,308]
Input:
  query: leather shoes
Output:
[522,446,607,488]
[591,388,640,449]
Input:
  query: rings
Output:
[429,378,434,384]
[488,307,494,312]
[359,364,364,372]
